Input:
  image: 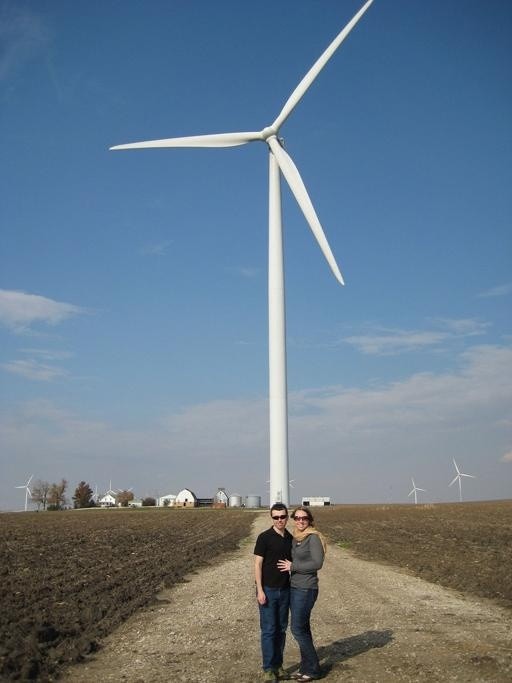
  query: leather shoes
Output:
[297,675,313,682]
[290,672,303,680]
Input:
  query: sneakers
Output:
[264,671,277,682]
[275,666,290,679]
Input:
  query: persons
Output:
[253,503,294,683]
[276,506,326,683]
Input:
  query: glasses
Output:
[272,515,287,519]
[294,516,309,521]
